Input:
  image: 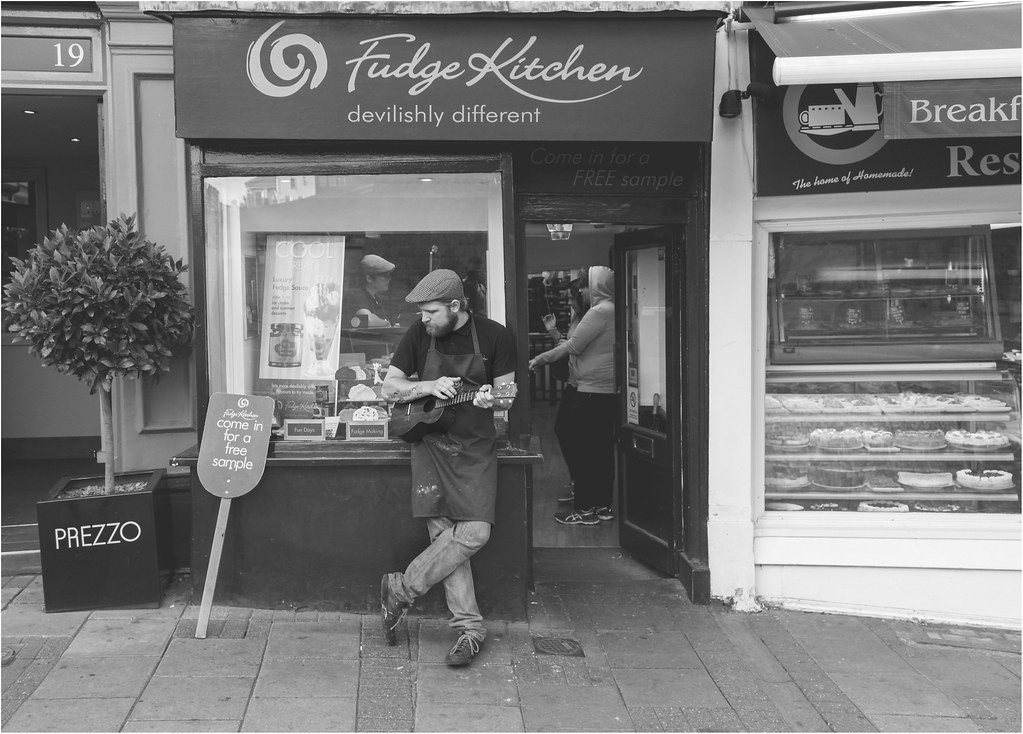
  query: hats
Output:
[360,254,395,275]
[402,268,465,303]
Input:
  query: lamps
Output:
[546,224,574,241]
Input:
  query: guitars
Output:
[391,381,518,443]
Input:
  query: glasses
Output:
[370,271,392,281]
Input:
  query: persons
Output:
[381,269,520,669]
[529,265,616,525]
[541,277,590,503]
[341,255,395,327]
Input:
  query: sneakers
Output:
[555,478,575,503]
[555,500,615,525]
[443,631,485,665]
[378,569,407,646]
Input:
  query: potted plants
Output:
[2,212,196,613]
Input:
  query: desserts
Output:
[767,393,1011,413]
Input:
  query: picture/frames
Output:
[346,420,390,441]
[284,418,327,441]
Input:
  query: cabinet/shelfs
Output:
[766,362,1023,513]
[769,223,1004,365]
[527,277,570,408]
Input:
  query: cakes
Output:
[766,428,1016,514]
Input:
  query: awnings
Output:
[742,1,1021,86]
